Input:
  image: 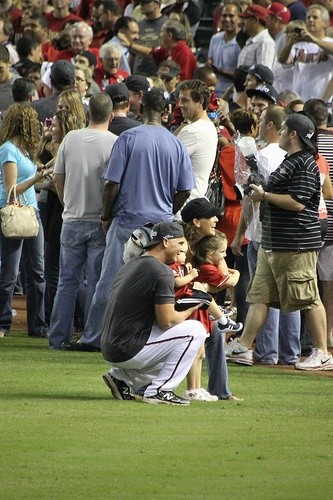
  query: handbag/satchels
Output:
[1,184,41,241]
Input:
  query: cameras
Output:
[233,173,264,200]
[293,28,304,36]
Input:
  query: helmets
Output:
[238,63,275,86]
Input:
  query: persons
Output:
[2,0,333,406]
[222,114,331,374]
[98,219,208,404]
[46,92,120,353]
[0,103,51,341]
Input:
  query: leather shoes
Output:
[61,341,98,352]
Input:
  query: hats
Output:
[78,50,97,66]
[156,60,181,78]
[180,197,223,222]
[49,60,75,86]
[237,5,270,20]
[286,113,316,153]
[265,2,290,24]
[142,221,183,250]
[246,83,279,104]
[105,83,129,106]
[123,74,151,92]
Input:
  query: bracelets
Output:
[261,191,267,200]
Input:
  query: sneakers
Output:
[218,307,243,332]
[294,349,333,371]
[226,337,253,366]
[185,387,219,402]
[103,372,135,400]
[143,390,191,406]
